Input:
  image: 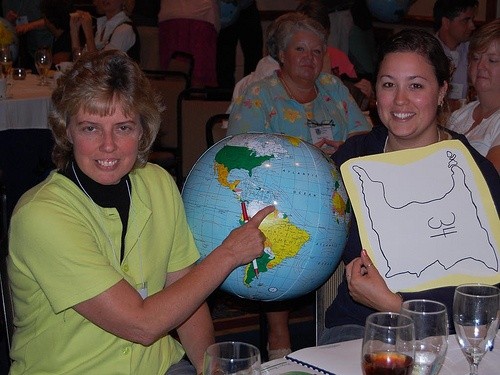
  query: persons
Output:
[324,29,500,343]
[8,52,274,375]
[226,14,373,361]
[158,0,500,177]
[0,0,141,70]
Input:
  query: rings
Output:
[345,274,351,280]
[366,263,373,268]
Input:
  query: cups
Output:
[360,311,416,375]
[55,62,73,73]
[395,298,449,375]
[13,67,26,80]
[202,341,262,375]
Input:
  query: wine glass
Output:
[0,44,14,100]
[34,47,52,87]
[452,282,500,375]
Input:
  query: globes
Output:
[180,132,352,302]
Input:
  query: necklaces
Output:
[384,127,441,151]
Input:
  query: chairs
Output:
[141,51,229,192]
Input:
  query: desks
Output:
[227,329,500,375]
[0,68,57,131]
